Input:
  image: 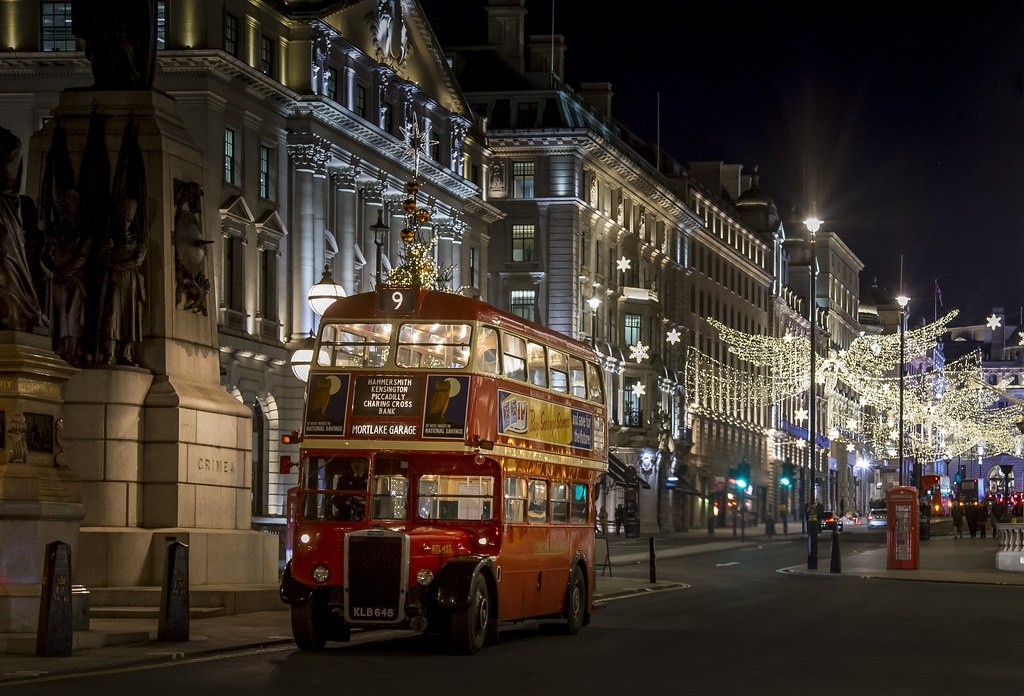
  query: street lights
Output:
[943,452,952,481]
[893,289,912,486]
[801,198,823,573]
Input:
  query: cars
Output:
[983,493,1024,505]
[866,509,888,529]
[820,513,843,531]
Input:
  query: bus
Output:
[921,475,950,516]
[960,478,984,506]
[278,288,609,653]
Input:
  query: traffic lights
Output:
[782,458,793,487]
[736,462,749,491]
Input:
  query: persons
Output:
[41,182,147,367]
[595,506,601,532]
[0,125,51,330]
[869,498,874,511]
[336,459,368,518]
[805,497,824,534]
[920,499,925,516]
[598,506,608,535]
[951,491,1024,540]
[614,502,633,535]
